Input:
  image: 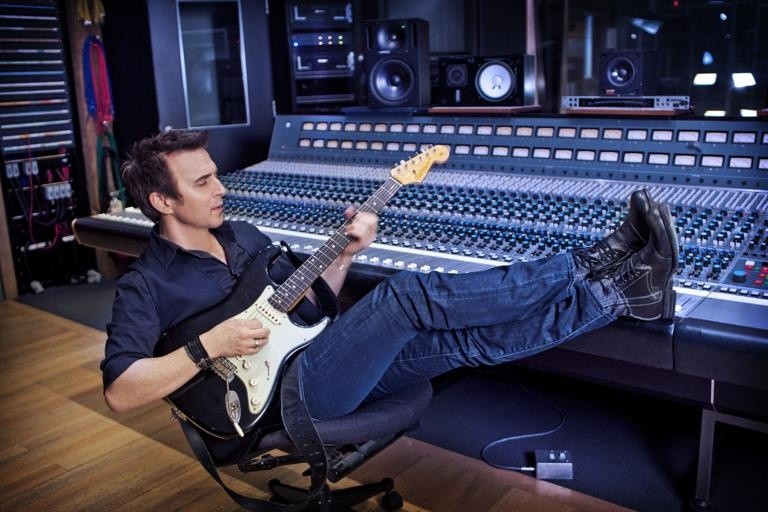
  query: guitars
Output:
[152,144,450,442]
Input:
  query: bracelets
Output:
[184,335,213,370]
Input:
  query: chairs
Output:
[105,322,435,512]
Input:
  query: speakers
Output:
[437,55,534,106]
[362,17,432,109]
[599,48,644,97]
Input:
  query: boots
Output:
[575,188,679,324]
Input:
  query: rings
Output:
[255,339,261,348]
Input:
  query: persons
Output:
[100,129,682,421]
